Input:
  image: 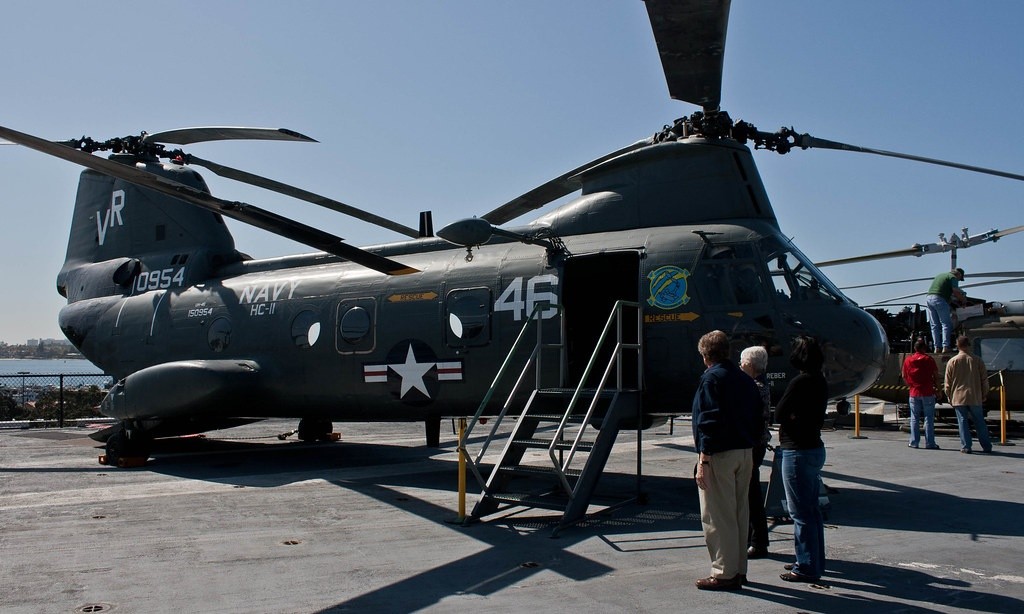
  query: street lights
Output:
[17,371,30,408]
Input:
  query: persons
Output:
[944,336,992,453]
[837,398,851,426]
[692,329,762,591]
[901,340,942,448]
[926,268,967,354]
[737,346,771,557]
[774,336,826,583]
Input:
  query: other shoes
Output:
[785,563,825,575]
[933,347,942,353]
[779,570,822,583]
[942,346,953,353]
[960,447,972,454]
[908,443,918,448]
[747,545,769,560]
[984,447,992,453]
[927,443,940,449]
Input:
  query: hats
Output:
[956,268,965,281]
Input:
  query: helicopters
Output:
[771,225,1024,416]
[0,0,1024,539]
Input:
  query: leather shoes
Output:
[695,575,741,593]
[737,573,748,585]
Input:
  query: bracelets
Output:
[699,461,709,464]
